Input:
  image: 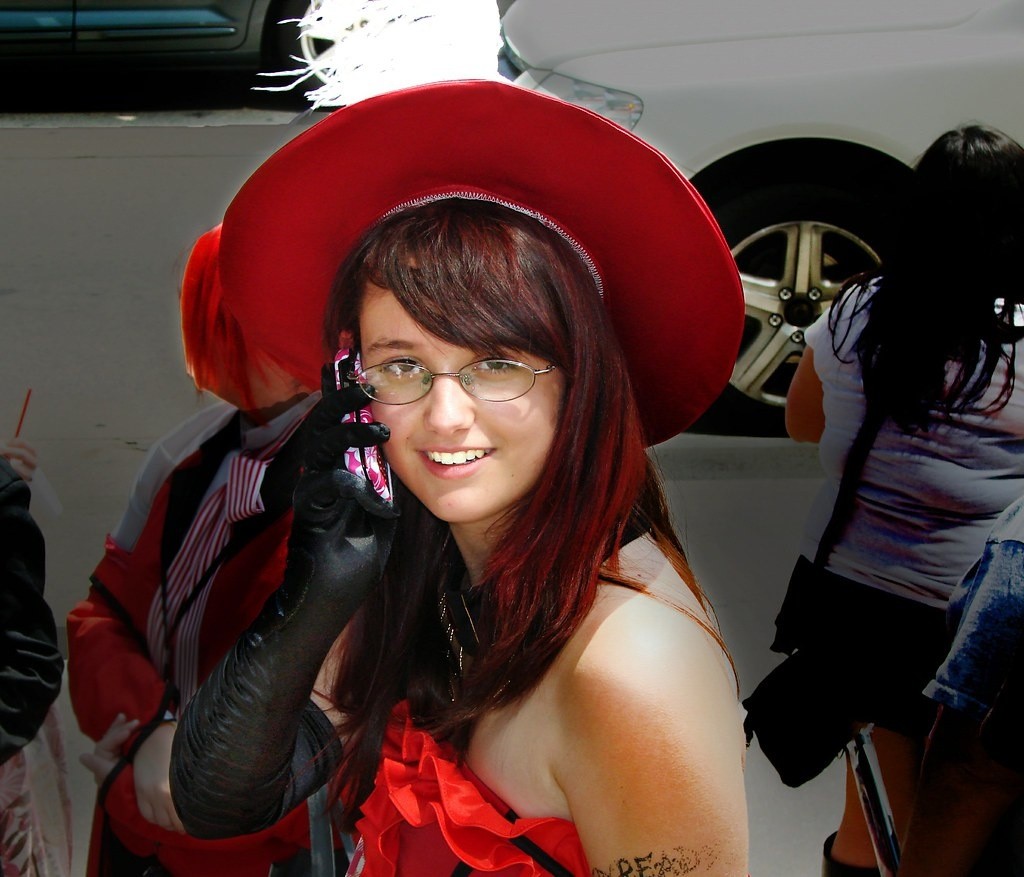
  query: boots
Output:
[815,827,889,876]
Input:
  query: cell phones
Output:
[332,348,398,516]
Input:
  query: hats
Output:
[214,73,743,456]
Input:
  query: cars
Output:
[496,2,1024,438]
[0,2,521,107]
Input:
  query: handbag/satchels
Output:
[738,627,887,792]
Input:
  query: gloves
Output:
[153,380,400,841]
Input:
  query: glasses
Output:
[339,352,563,408]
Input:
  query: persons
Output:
[57,210,396,876]
[2,440,71,876]
[780,120,1024,876]
[128,78,760,877]
